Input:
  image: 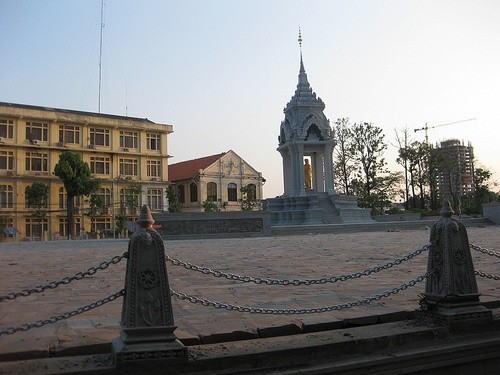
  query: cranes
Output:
[414,117,477,148]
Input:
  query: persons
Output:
[303,158,312,191]
